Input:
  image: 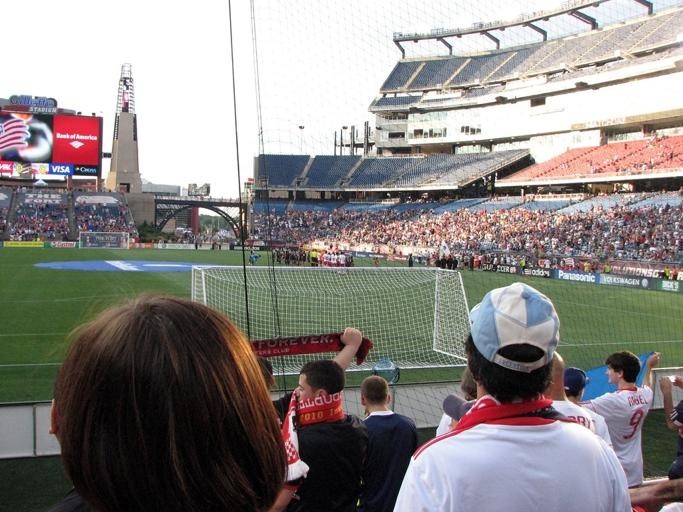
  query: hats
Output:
[564,367,589,390]
[470,282,560,374]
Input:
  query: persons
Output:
[439,392,479,432]
[266,359,372,512]
[392,281,632,512]
[359,375,417,512]
[48,292,290,512]
[247,180,682,283]
[556,131,682,178]
[0,182,136,247]
[172,226,241,250]
[250,325,363,433]
[434,365,478,438]
[543,351,682,512]
